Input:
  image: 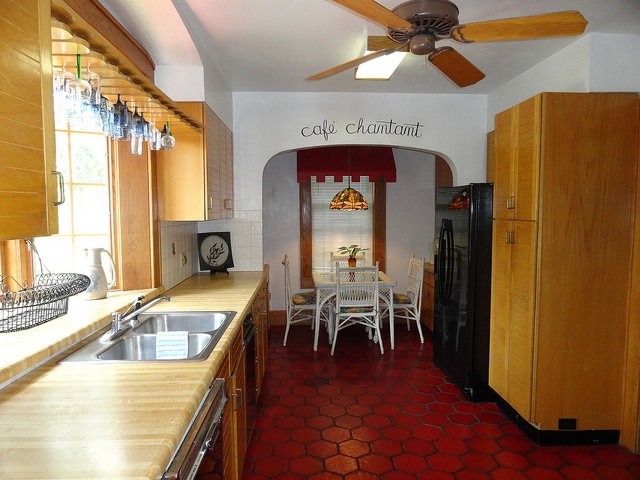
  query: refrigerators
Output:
[432,182,493,404]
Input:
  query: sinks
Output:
[131,313,227,332]
[96,333,212,360]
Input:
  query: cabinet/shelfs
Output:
[0,0,66,243]
[251,275,269,397]
[212,328,247,479]
[488,92,640,445]
[0,238,90,334]
[155,102,233,223]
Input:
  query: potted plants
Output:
[336,244,370,282]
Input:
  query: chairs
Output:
[282,255,334,347]
[329,261,385,355]
[330,251,366,274]
[367,259,426,346]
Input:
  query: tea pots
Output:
[79,247,117,301]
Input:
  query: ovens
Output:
[161,377,230,480]
[243,311,258,446]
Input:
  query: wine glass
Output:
[52,54,176,156]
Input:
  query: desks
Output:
[312,269,397,351]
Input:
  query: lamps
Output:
[328,175,370,211]
[353,36,409,81]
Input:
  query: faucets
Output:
[111,295,171,331]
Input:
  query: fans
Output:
[303,0,589,89]
